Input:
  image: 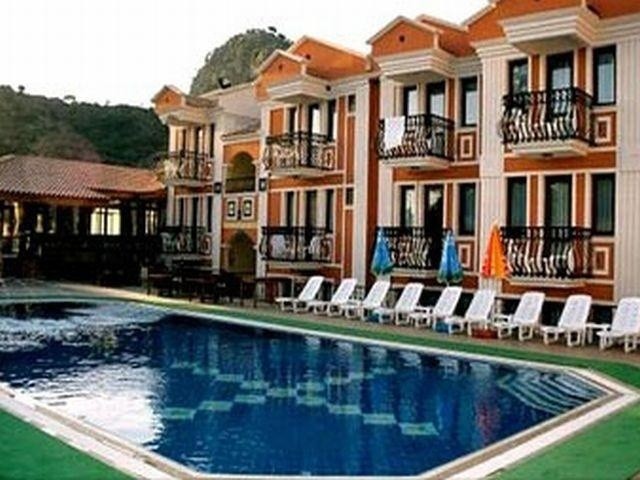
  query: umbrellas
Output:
[371,228,392,277]
[472,384,504,449]
[436,228,462,290]
[481,220,509,288]
[428,388,459,436]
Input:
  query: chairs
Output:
[410,284,465,333]
[338,278,393,322]
[444,288,500,337]
[597,295,640,354]
[372,281,429,327]
[538,293,594,348]
[275,274,326,313]
[308,278,361,318]
[492,290,547,342]
